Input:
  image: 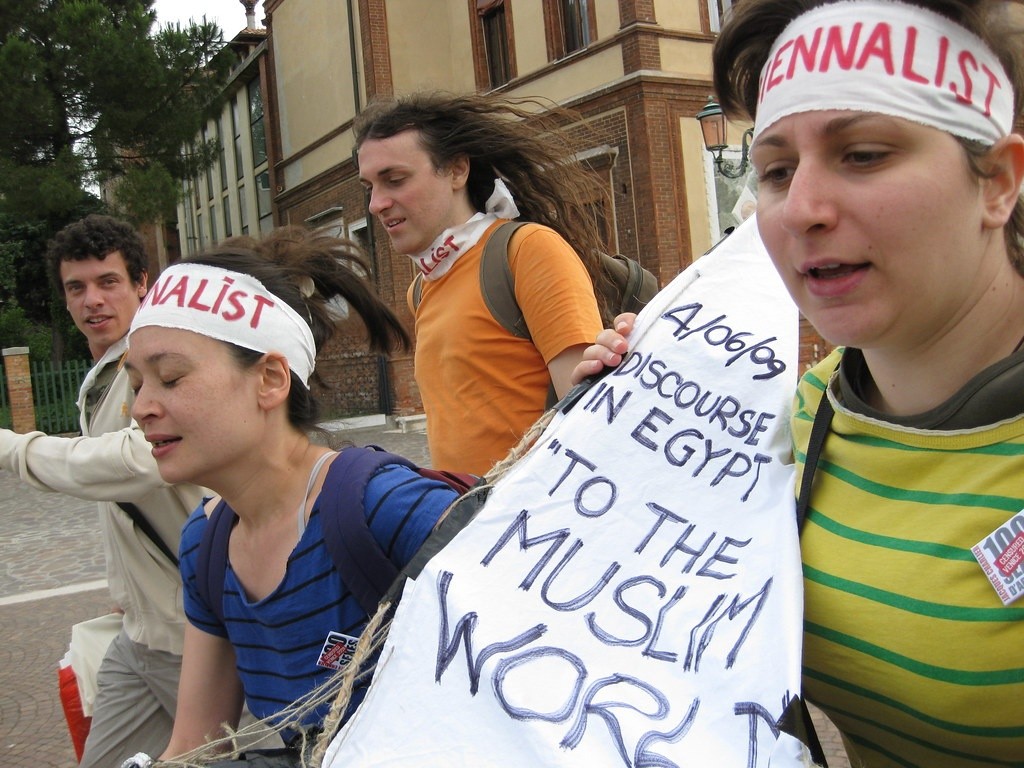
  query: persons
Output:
[0,213,217,768]
[123,229,461,768]
[354,94,606,480]
[569,0,1024,768]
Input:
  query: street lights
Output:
[697,95,755,179]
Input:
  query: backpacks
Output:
[412,222,658,411]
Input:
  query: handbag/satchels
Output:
[58,611,126,764]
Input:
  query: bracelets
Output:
[121,751,153,768]
